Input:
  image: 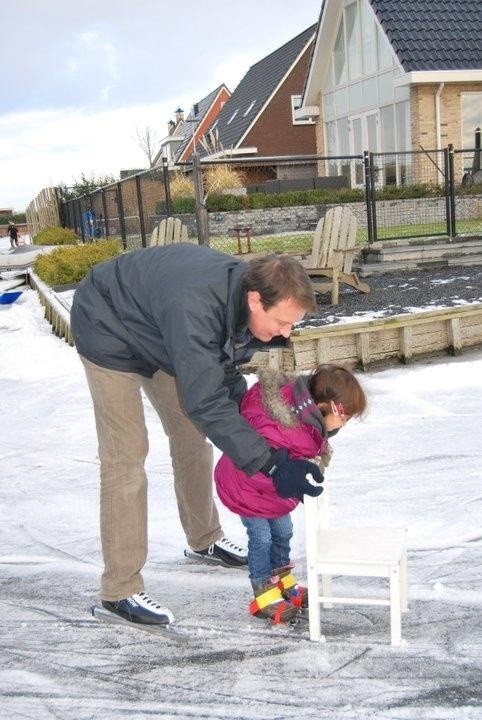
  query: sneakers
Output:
[102,591,175,625]
[194,537,248,566]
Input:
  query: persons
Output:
[5,219,20,249]
[70,242,325,627]
[215,361,366,624]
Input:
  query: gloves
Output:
[261,448,323,503]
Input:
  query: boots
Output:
[251,576,298,621]
[272,563,308,607]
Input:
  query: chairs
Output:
[304,457,407,646]
[149,218,189,247]
[301,206,370,303]
